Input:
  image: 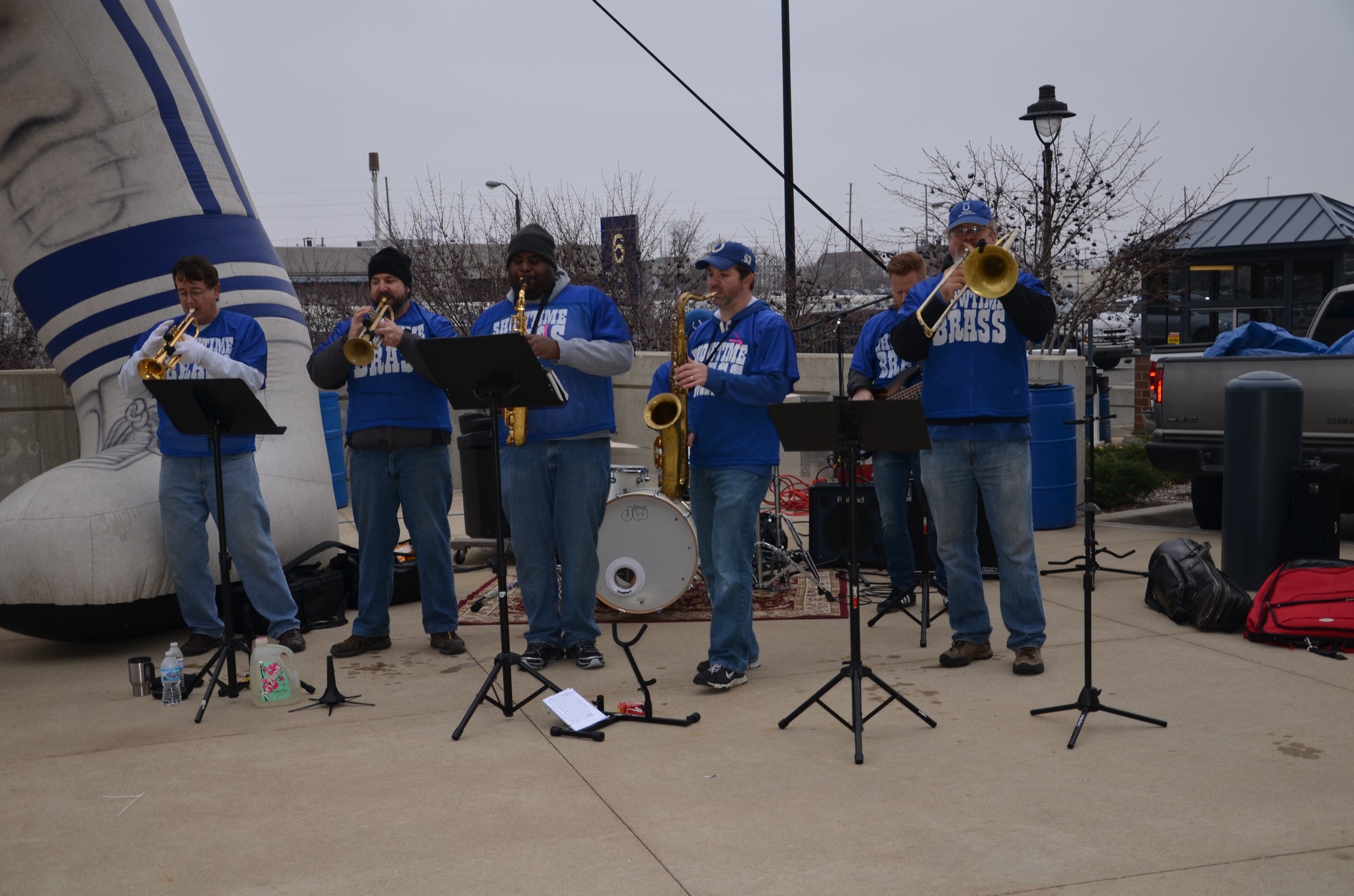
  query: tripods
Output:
[1029,363,1169,748]
[138,377,314,724]
[394,334,565,742]
[754,403,955,769]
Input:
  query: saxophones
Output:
[503,279,530,448]
[643,290,720,500]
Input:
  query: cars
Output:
[838,288,859,295]
[858,288,873,295]
[1078,293,1253,370]
[874,288,886,295]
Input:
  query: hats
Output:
[505,224,561,274]
[368,246,411,289]
[946,201,994,233]
[694,242,757,275]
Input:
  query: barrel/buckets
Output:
[319,391,349,509]
[1029,383,1079,530]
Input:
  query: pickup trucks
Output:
[1142,283,1354,530]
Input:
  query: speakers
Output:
[806,482,931,571]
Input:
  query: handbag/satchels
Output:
[1145,538,1251,632]
[1242,559,1353,661]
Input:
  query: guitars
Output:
[846,364,924,462]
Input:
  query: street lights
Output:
[368,152,381,259]
[1019,83,1076,293]
[485,179,521,231]
[927,228,942,276]
[900,226,918,254]
[1266,176,1271,197]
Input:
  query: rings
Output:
[690,376,694,381]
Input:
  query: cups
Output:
[128,655,155,697]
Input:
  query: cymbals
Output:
[609,439,652,449]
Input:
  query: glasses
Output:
[174,285,210,302]
[953,225,986,238]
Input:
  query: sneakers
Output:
[333,630,391,659]
[1011,644,1044,676]
[565,640,605,669]
[692,663,747,690]
[180,633,224,656]
[696,655,761,673]
[941,594,949,613]
[430,629,466,655]
[939,639,993,666]
[885,587,917,619]
[519,640,565,671]
[279,629,307,653]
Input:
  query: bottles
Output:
[168,642,186,693]
[159,651,182,706]
[249,636,303,708]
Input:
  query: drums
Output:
[587,486,701,614]
[606,463,650,503]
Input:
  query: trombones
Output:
[914,224,1021,340]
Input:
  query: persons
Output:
[889,200,1057,676]
[473,224,634,670]
[847,251,953,616]
[312,246,467,656]
[648,242,799,688]
[116,257,306,656]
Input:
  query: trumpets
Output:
[343,295,395,368]
[137,305,204,382]
